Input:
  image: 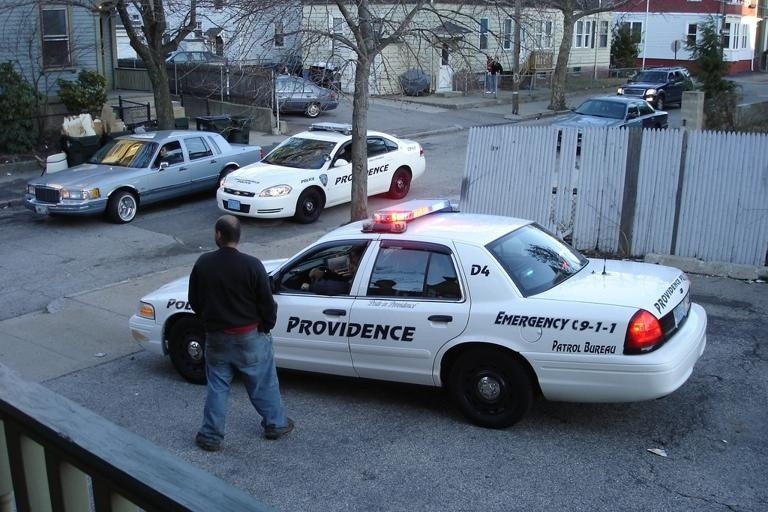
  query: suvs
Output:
[616,66,694,110]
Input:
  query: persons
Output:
[301,247,364,296]
[490,57,503,93]
[485,56,492,94]
[155,146,171,168]
[189,215,294,451]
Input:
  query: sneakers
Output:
[266,417,295,440]
[195,433,221,452]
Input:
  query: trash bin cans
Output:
[194,115,231,142]
[175,117,190,130]
[61,135,100,168]
[231,114,251,144]
[103,131,132,141]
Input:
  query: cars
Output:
[551,93,668,153]
[165,51,231,72]
[129,195,708,430]
[264,54,302,73]
[216,122,426,222]
[273,74,339,119]
[22,129,267,223]
